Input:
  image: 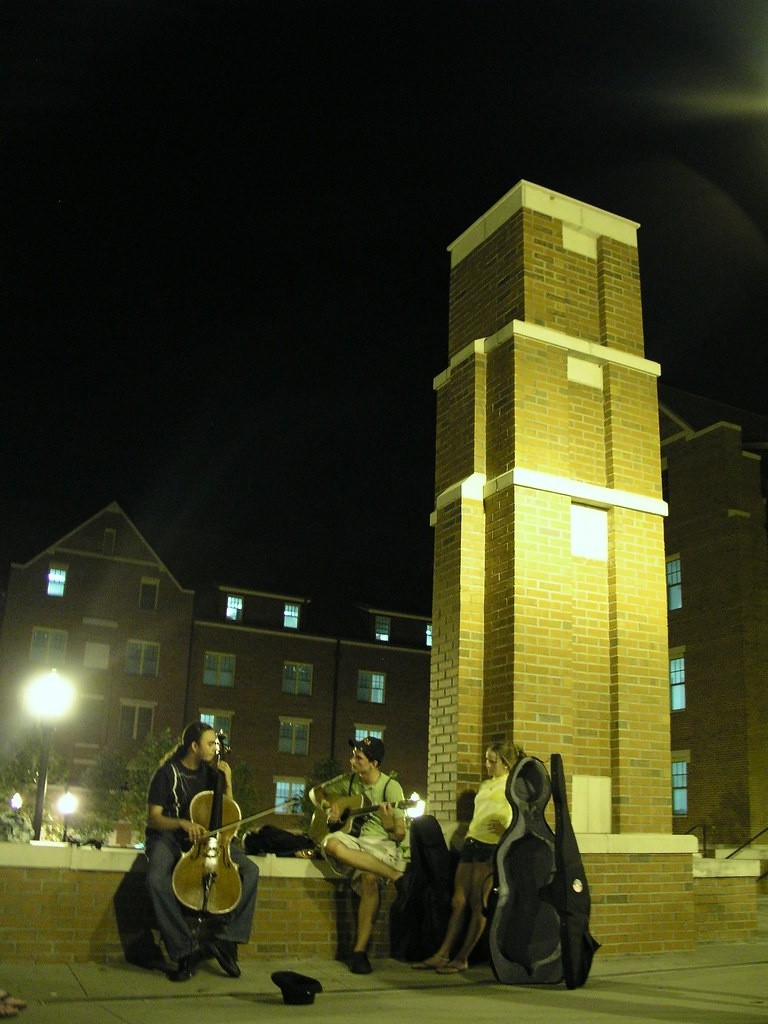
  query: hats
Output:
[348,737,384,765]
[270,970,323,1004]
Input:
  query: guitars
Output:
[308,792,420,845]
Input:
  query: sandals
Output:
[437,960,469,973]
[411,951,450,970]
[0,989,27,1020]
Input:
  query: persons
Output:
[0,989,27,1016]
[411,741,520,974]
[309,736,412,975]
[145,723,260,979]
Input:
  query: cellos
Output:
[170,728,245,979]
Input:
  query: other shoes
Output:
[393,872,415,913]
[210,939,240,976]
[349,950,371,973]
[176,947,203,982]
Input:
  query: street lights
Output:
[58,785,78,841]
[23,669,73,840]
